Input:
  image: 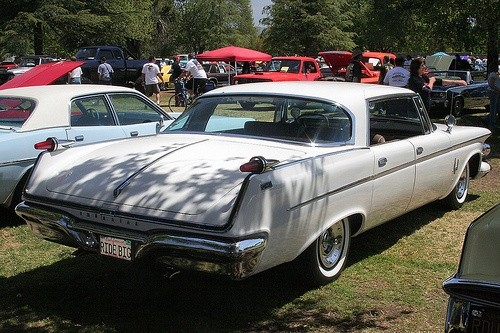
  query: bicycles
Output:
[168,79,218,112]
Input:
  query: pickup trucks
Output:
[72,46,162,84]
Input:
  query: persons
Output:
[344,52,500,126]
[98,56,114,84]
[142,56,163,110]
[57,55,83,84]
[161,53,265,106]
[294,63,303,72]
[339,116,385,144]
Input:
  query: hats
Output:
[188,54,195,59]
[351,48,362,58]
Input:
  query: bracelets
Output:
[378,136,383,143]
[178,77,180,80]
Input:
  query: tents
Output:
[193,45,275,93]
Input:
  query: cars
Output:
[425,80,498,161]
[232,56,322,82]
[442,203,500,332]
[0,57,53,83]
[0,83,256,219]
[15,81,493,289]
[170,54,190,66]
[319,50,396,83]
[0,60,87,127]
[187,62,238,82]
